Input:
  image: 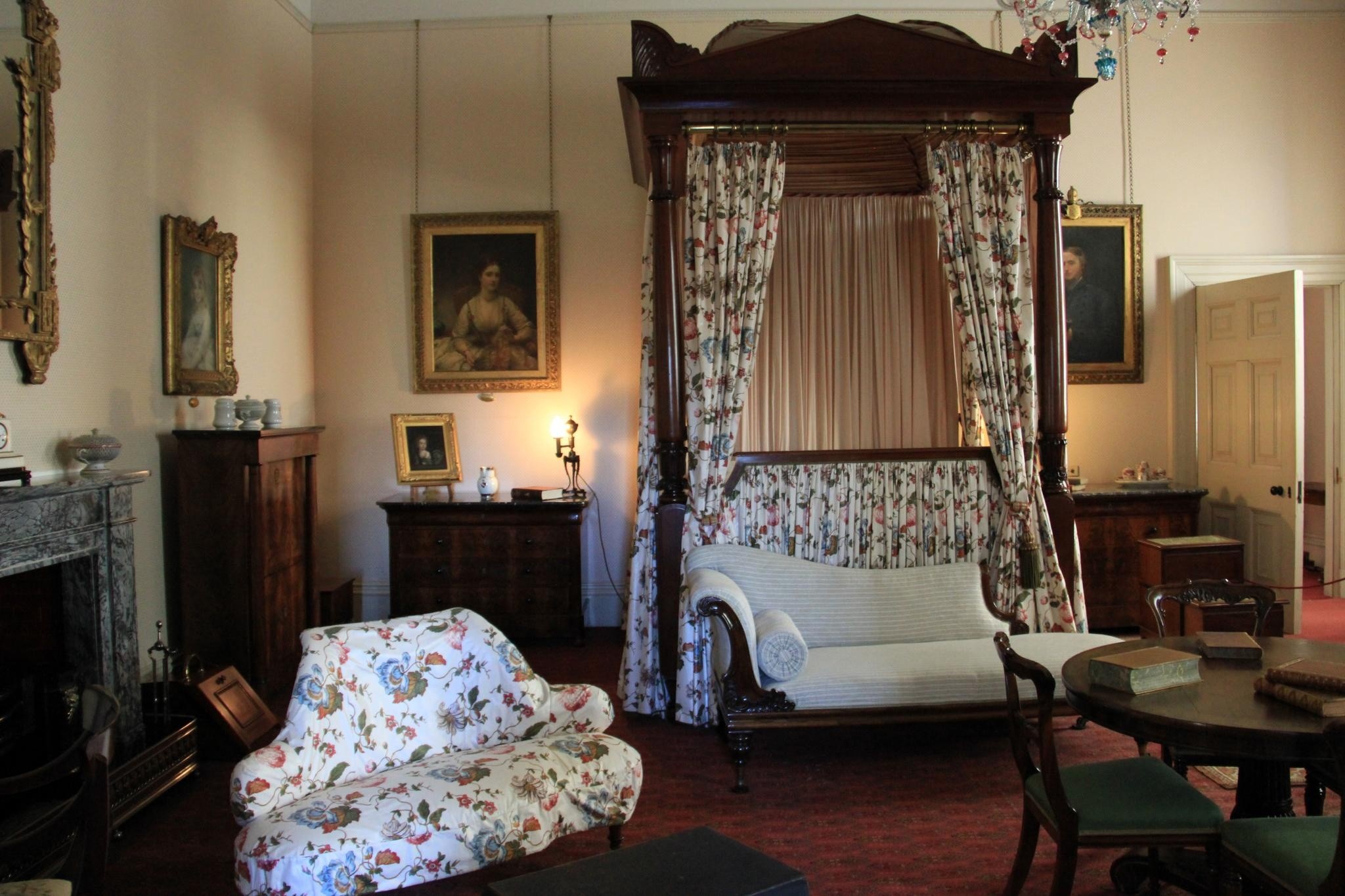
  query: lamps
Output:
[1013,0,1200,80]
[549,415,586,498]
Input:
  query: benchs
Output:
[685,545,1126,793]
[229,607,643,896]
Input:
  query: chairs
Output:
[993,578,1345,896]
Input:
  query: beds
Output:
[618,14,1088,728]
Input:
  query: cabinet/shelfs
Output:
[171,425,326,712]
[1071,483,1289,637]
[376,492,595,646]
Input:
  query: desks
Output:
[1062,638,1345,896]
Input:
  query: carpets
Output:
[1195,766,1307,789]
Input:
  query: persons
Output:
[181,269,216,370]
[409,434,433,466]
[433,253,538,372]
[1063,246,1118,364]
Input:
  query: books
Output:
[1253,656,1345,718]
[1088,645,1206,695]
[511,486,563,502]
[1196,631,1263,660]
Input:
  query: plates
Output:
[1115,477,1171,483]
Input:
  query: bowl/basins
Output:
[234,395,268,431]
[67,429,123,475]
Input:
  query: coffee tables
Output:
[487,828,810,896]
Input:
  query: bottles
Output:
[477,467,498,499]
[261,398,283,429]
[212,398,238,431]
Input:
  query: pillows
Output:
[755,609,808,680]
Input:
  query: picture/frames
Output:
[0,0,62,385]
[1061,204,1144,384]
[410,210,561,394]
[390,413,462,486]
[161,214,239,397]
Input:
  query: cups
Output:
[1122,460,1166,481]
